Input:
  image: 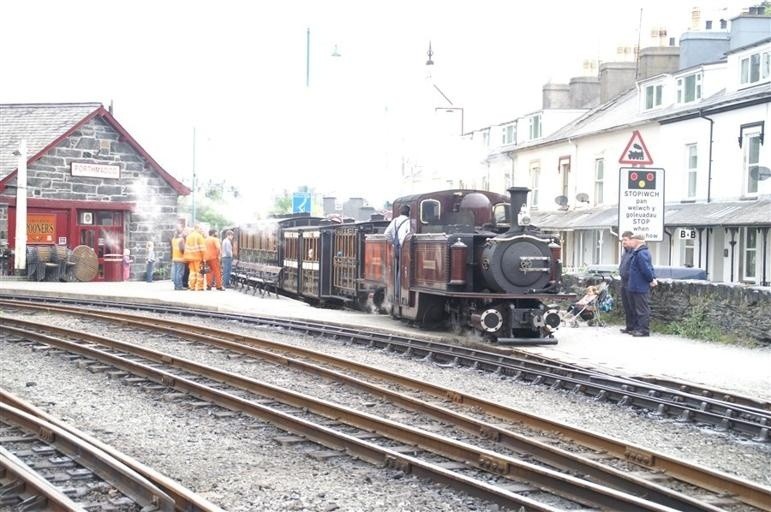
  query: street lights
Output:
[12,148,30,275]
[303,27,343,89]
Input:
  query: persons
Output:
[382,204,413,304]
[221,229,236,289]
[627,234,660,337]
[181,224,190,287]
[618,231,637,334]
[170,223,189,290]
[203,229,226,291]
[182,225,205,290]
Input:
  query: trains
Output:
[217,182,566,350]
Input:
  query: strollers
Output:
[558,273,618,328]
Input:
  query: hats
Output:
[631,230,647,240]
[225,230,236,236]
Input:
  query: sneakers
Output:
[173,285,236,291]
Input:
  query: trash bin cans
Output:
[103,254,124,282]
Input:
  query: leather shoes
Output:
[620,327,650,337]
